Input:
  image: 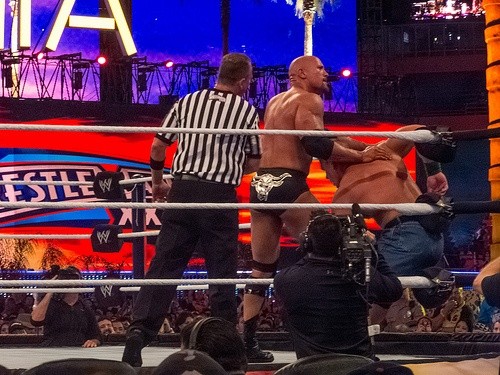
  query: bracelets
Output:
[424,162,441,176]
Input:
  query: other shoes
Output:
[123,328,143,366]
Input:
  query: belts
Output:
[383,214,413,230]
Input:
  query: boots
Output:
[242,313,274,363]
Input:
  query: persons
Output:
[30,266,105,348]
[273,209,403,361]
[122,52,264,367]
[0,258,500,374]
[319,124,450,325]
[239,55,392,363]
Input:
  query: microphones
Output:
[351,203,367,232]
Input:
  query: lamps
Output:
[1,44,352,101]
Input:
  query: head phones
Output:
[302,214,350,250]
